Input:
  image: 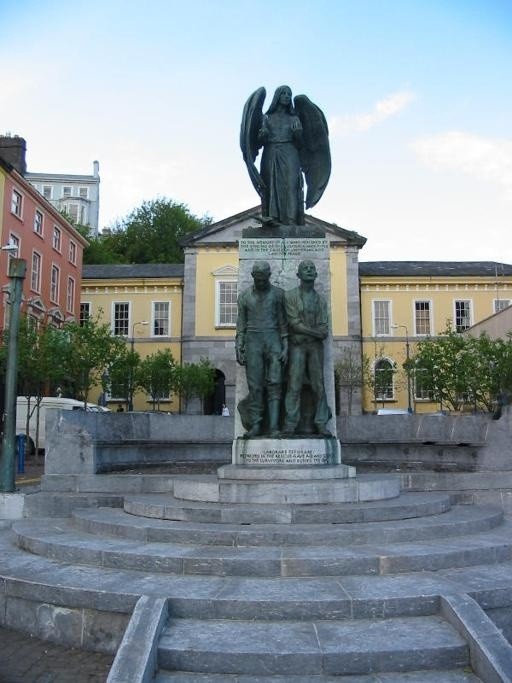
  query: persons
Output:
[255,84,308,226]
[280,258,337,439]
[221,402,229,416]
[117,404,124,412]
[234,258,289,439]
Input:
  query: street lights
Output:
[391,324,411,408]
[2,239,28,492]
[129,320,150,401]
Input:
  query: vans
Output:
[2,396,107,456]
